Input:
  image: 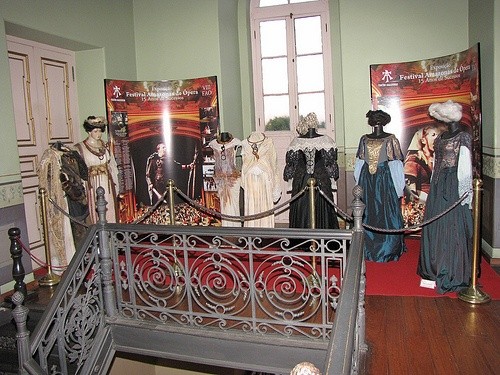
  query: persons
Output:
[209,132,242,228]
[73,115,119,224]
[146,141,194,207]
[38,141,89,276]
[416,100,475,294]
[240,131,282,229]
[401,126,439,235]
[283,112,350,251]
[353,110,407,264]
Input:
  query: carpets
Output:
[32,237,500,300]
